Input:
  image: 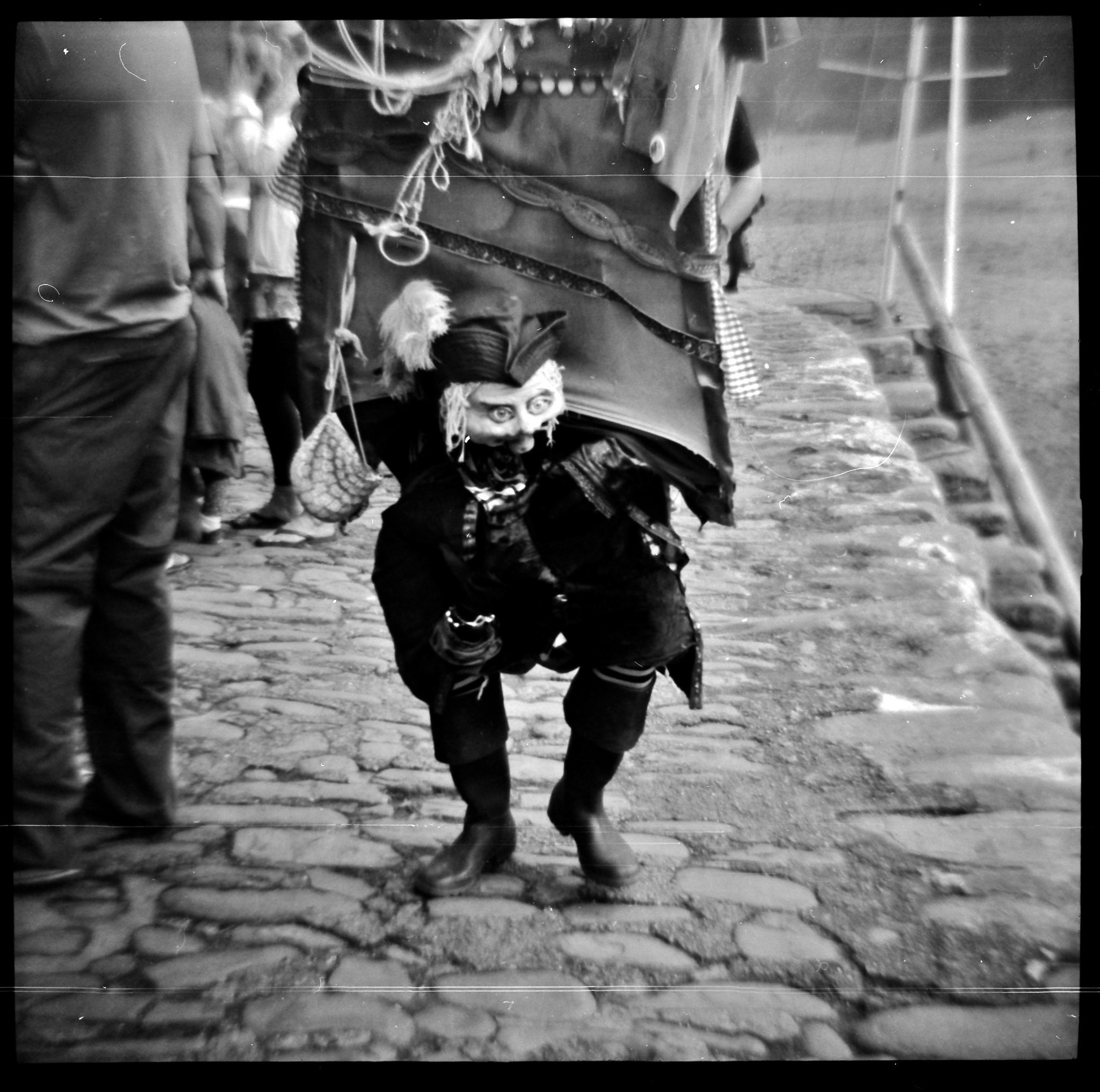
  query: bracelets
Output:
[204,261,225,270]
[718,218,734,242]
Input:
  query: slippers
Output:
[165,552,195,574]
[254,523,340,549]
[229,511,287,530]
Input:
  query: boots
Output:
[411,745,518,897]
[545,728,643,887]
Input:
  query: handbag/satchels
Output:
[289,411,385,527]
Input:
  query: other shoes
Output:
[172,520,226,545]
[14,779,175,897]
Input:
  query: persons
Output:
[268,17,766,902]
[14,21,229,883]
[173,18,769,546]
[379,279,570,463]
